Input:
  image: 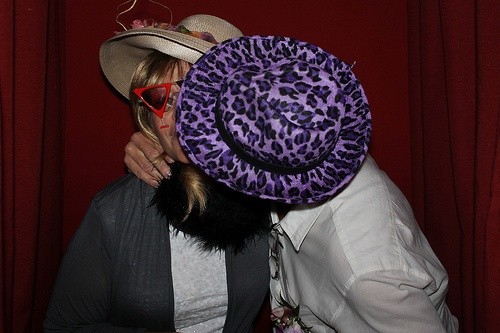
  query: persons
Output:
[41,13,272,333]
[124,34,459,333]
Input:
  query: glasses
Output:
[133,79,188,129]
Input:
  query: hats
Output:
[174,35,373,206]
[99,13,243,102]
